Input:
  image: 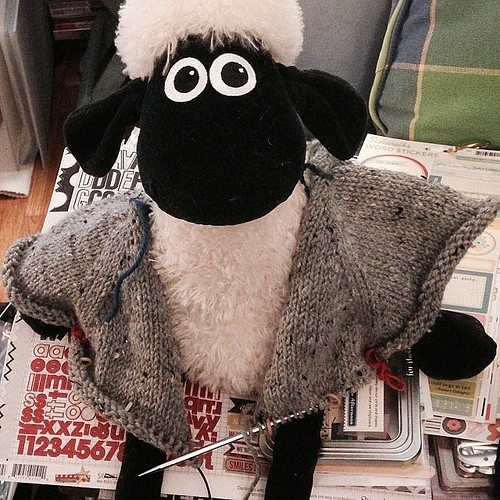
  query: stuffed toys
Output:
[1,0,500,500]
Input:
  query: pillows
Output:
[368,1,500,157]
